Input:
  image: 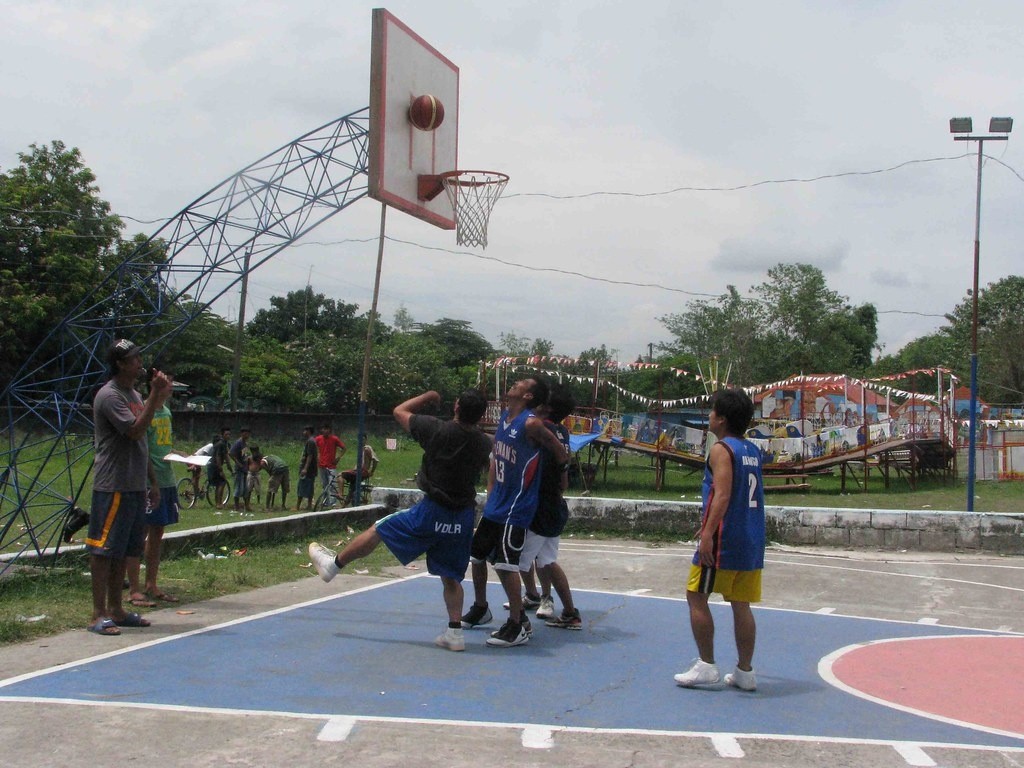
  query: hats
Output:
[213,435,223,440]
[108,339,146,364]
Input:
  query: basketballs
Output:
[408,93,445,132]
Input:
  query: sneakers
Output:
[490,620,533,638]
[536,596,555,619]
[309,542,342,583]
[460,602,492,630]
[673,657,720,686]
[544,608,583,630]
[722,666,758,691]
[504,594,540,609]
[485,626,529,647]
[435,631,466,650]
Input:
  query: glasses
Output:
[455,395,460,400]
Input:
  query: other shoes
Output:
[63,506,87,542]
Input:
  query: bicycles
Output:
[314,467,372,512]
[176,465,231,510]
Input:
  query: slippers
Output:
[87,619,121,636]
[151,590,179,602]
[127,593,156,607]
[114,612,150,628]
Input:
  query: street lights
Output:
[950,117,1014,512]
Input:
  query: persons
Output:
[126,361,179,607]
[228,425,251,514]
[516,381,582,630]
[313,422,346,509]
[674,387,765,690]
[309,389,492,650]
[206,427,234,510]
[253,451,290,512]
[186,433,225,498]
[246,444,261,506]
[461,376,569,648]
[330,431,379,508]
[84,338,168,636]
[290,424,318,511]
[502,555,554,619]
